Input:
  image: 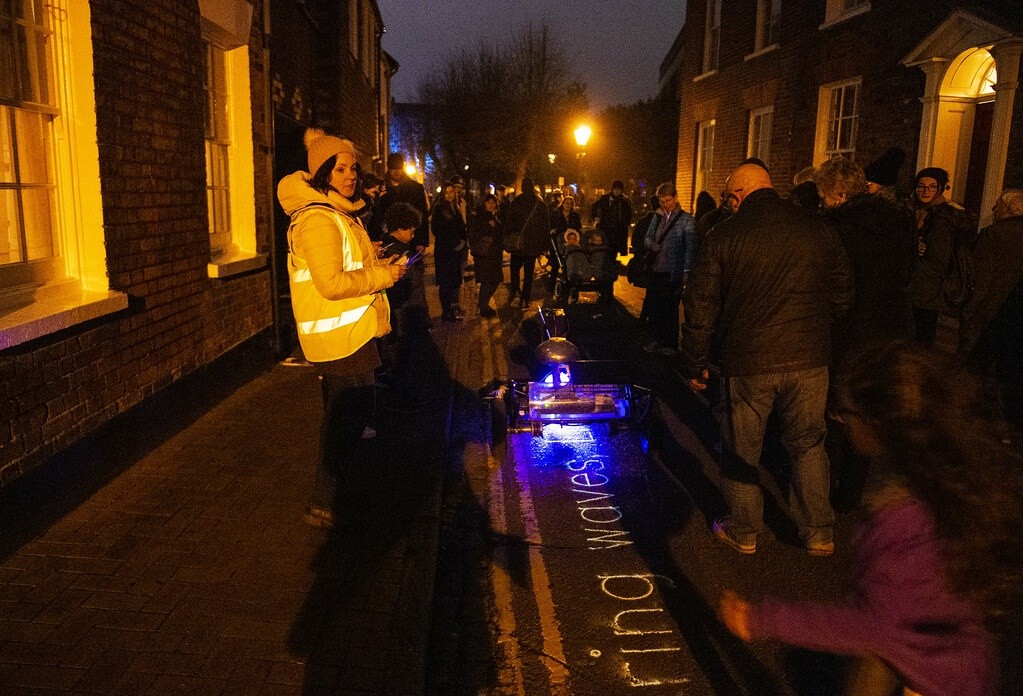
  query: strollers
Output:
[550,229,612,302]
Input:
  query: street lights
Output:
[574,125,591,207]
[548,153,556,192]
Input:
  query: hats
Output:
[451,176,463,185]
[303,128,356,178]
[863,146,904,185]
[914,167,949,196]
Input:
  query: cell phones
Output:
[384,242,394,249]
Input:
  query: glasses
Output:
[916,183,937,192]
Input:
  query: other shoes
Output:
[521,300,532,311]
[454,304,465,315]
[477,303,496,317]
[441,313,464,323]
[303,501,368,538]
[361,427,376,439]
[550,271,558,283]
[510,294,520,307]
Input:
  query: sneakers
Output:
[807,540,834,557]
[713,516,755,554]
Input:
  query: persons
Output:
[794,164,822,211]
[381,153,436,340]
[644,182,695,352]
[430,183,468,324]
[695,190,717,221]
[504,177,550,308]
[349,162,386,232]
[372,158,386,180]
[554,190,564,203]
[680,155,855,555]
[468,195,504,317]
[720,472,1023,696]
[278,128,409,532]
[632,196,661,326]
[582,228,611,251]
[497,185,509,204]
[590,180,632,260]
[448,175,467,315]
[547,196,581,293]
[545,193,557,209]
[813,157,1023,525]
[377,202,434,411]
[485,184,496,195]
[564,227,585,252]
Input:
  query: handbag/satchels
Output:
[471,235,497,259]
[625,256,648,287]
[502,233,526,252]
